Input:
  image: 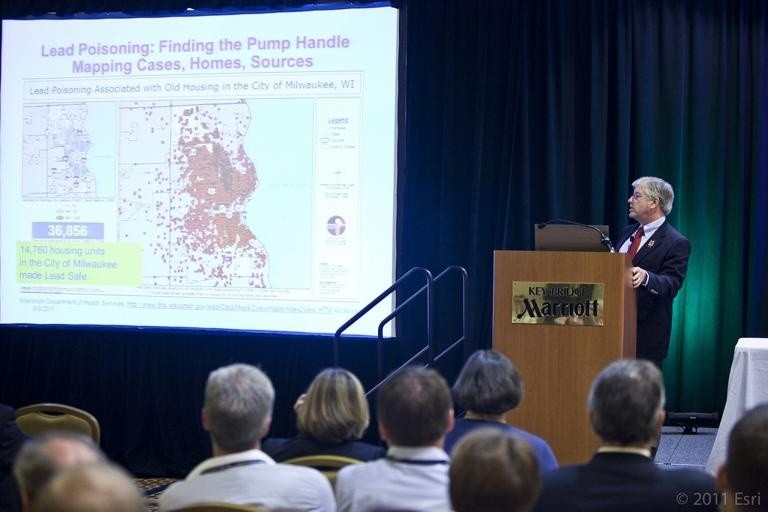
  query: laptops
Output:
[533,224,608,252]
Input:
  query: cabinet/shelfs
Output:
[492,251,638,468]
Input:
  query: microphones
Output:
[537,219,613,252]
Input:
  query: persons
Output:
[29,463,147,511]
[449,426,542,512]
[444,351,559,475]
[534,359,721,512]
[0,401,31,511]
[261,367,387,464]
[716,403,768,512]
[159,364,338,511]
[12,431,110,511]
[613,176,691,463]
[335,367,457,512]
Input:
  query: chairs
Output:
[15,402,177,496]
[279,455,364,495]
[170,503,262,512]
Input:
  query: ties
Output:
[627,226,645,262]
